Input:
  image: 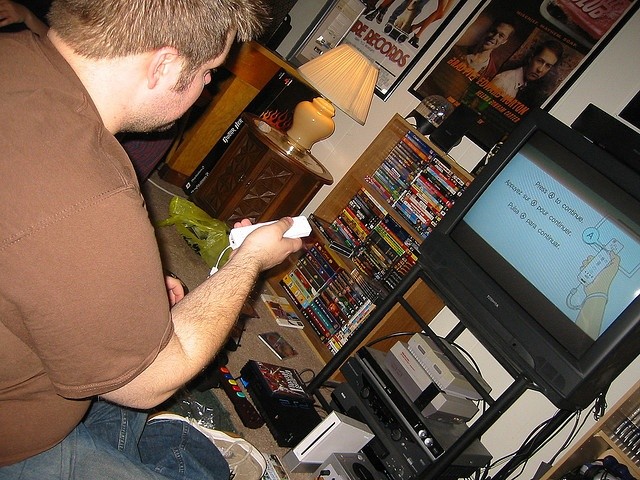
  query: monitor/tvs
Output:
[418,115,640,402]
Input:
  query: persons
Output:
[490,40,563,98]
[0,1,302,479]
[575,250,621,341]
[461,13,520,72]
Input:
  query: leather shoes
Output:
[398,32,409,43]
[385,16,396,34]
[365,10,378,21]
[376,10,386,24]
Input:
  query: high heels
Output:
[409,27,413,35]
[409,33,419,49]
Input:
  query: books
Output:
[364,129,470,240]
[309,190,421,304]
[257,241,376,361]
